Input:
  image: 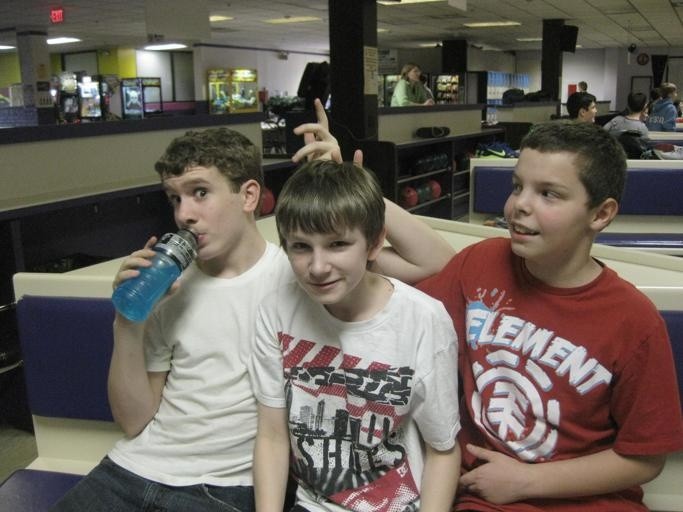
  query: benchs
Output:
[647,131,683,145]
[468,158,682,253]
[0,270,683,512]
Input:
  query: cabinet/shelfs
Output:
[485,70,530,105]
[453,132,502,218]
[377,138,455,217]
[431,75,460,104]
[383,74,402,107]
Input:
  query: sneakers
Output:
[476,144,520,158]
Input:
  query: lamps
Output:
[143,42,189,52]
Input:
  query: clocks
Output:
[636,53,649,65]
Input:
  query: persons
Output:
[248,159,462,512]
[568,92,597,123]
[673,99,683,118]
[603,90,649,136]
[646,83,678,132]
[390,63,435,107]
[641,88,662,123]
[331,118,682,512]
[578,82,597,102]
[57,99,456,512]
[419,75,435,100]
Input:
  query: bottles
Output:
[112,227,197,321]
